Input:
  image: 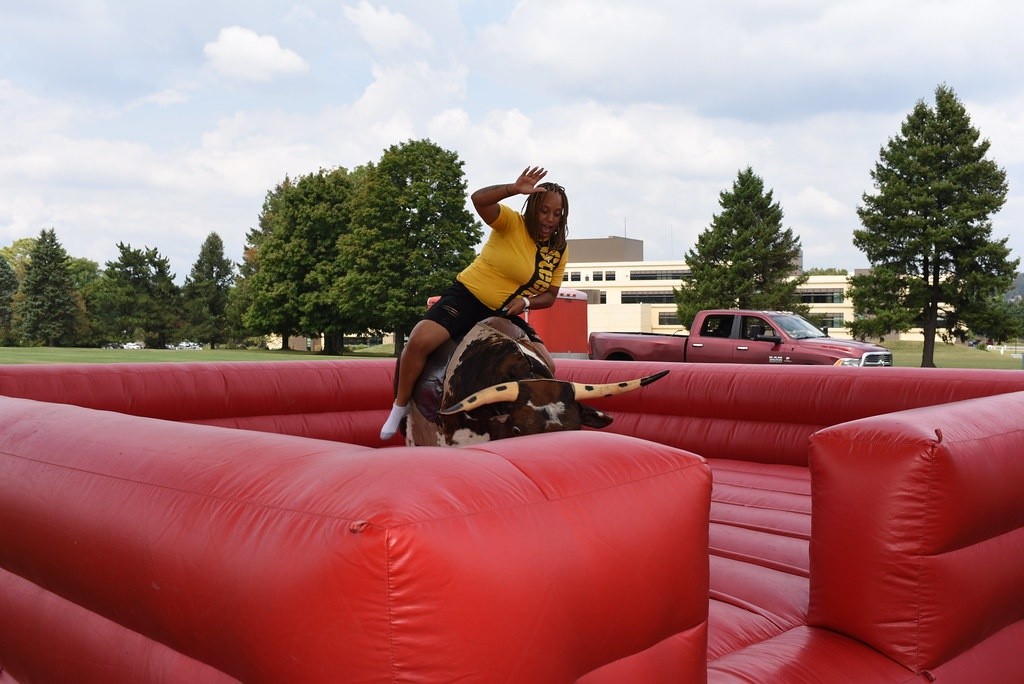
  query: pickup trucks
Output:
[588,309,895,369]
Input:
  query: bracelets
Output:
[522,297,530,313]
[506,184,512,194]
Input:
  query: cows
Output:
[403,314,671,448]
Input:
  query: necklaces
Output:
[536,240,551,263]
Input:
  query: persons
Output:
[380,166,567,441]
